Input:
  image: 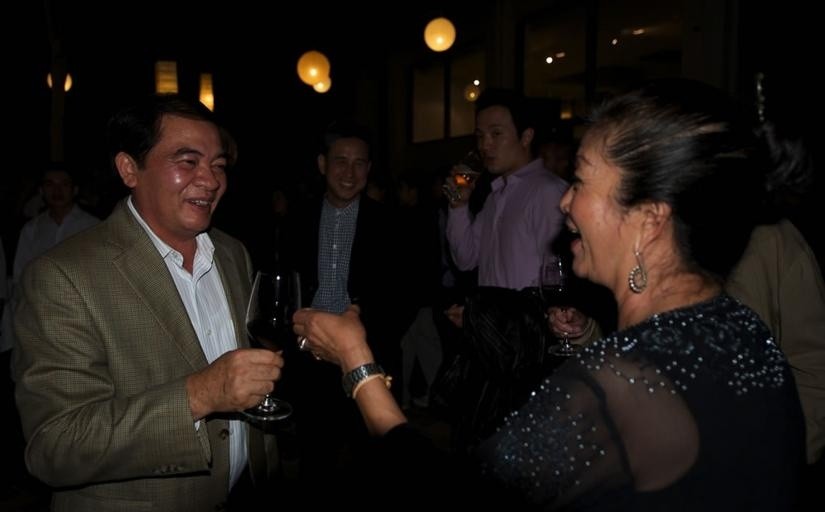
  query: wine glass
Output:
[238,266,303,421]
[441,147,486,203]
[538,255,588,357]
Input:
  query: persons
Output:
[0,84,823,512]
